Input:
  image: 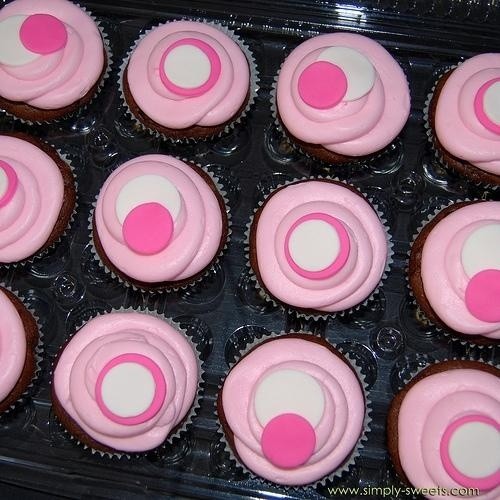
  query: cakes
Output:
[212,327,373,491]
[242,175,396,322]
[422,53,500,191]
[1,1,117,126]
[48,306,205,461]
[386,358,500,500]
[1,131,80,270]
[86,152,234,295]
[404,197,500,339]
[1,283,44,416]
[267,32,412,166]
[115,15,262,143]
